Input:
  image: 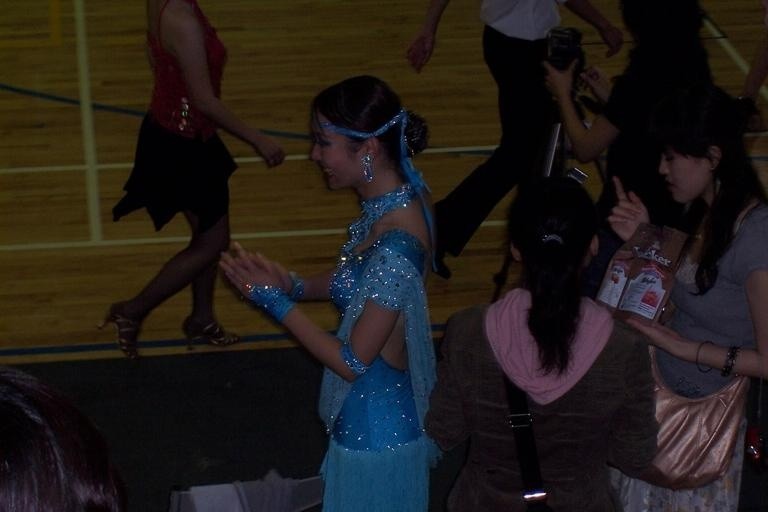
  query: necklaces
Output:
[342,182,429,248]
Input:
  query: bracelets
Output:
[289,268,304,300]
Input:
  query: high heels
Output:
[96,301,138,359]
[181,316,237,350]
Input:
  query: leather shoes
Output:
[431,258,451,279]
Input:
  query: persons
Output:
[401,1,768,511]
[218,76,443,510]
[0,368,130,512]
[97,1,285,361]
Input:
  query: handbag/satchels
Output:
[641,344,747,491]
